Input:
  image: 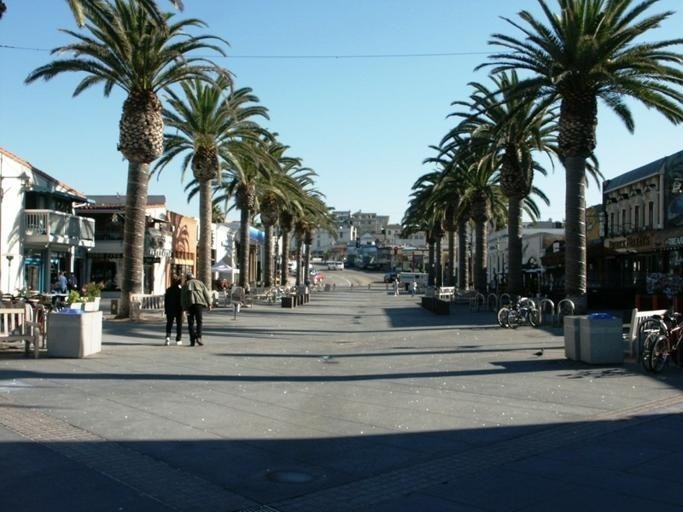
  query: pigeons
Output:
[532,347,545,357]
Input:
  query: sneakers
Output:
[176,341,182,345]
[190,339,205,348]
[164,338,170,346]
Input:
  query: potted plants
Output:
[63,280,106,312]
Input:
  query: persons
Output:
[162,279,184,346]
[56,272,67,295]
[181,272,213,347]
[392,277,399,296]
[69,272,77,291]
[409,279,417,297]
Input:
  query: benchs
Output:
[621,307,668,358]
[0,302,42,359]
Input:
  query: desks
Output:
[47,309,103,358]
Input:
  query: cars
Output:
[327,261,344,271]
[384,271,397,282]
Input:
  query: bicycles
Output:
[497,298,538,329]
[641,311,683,374]
